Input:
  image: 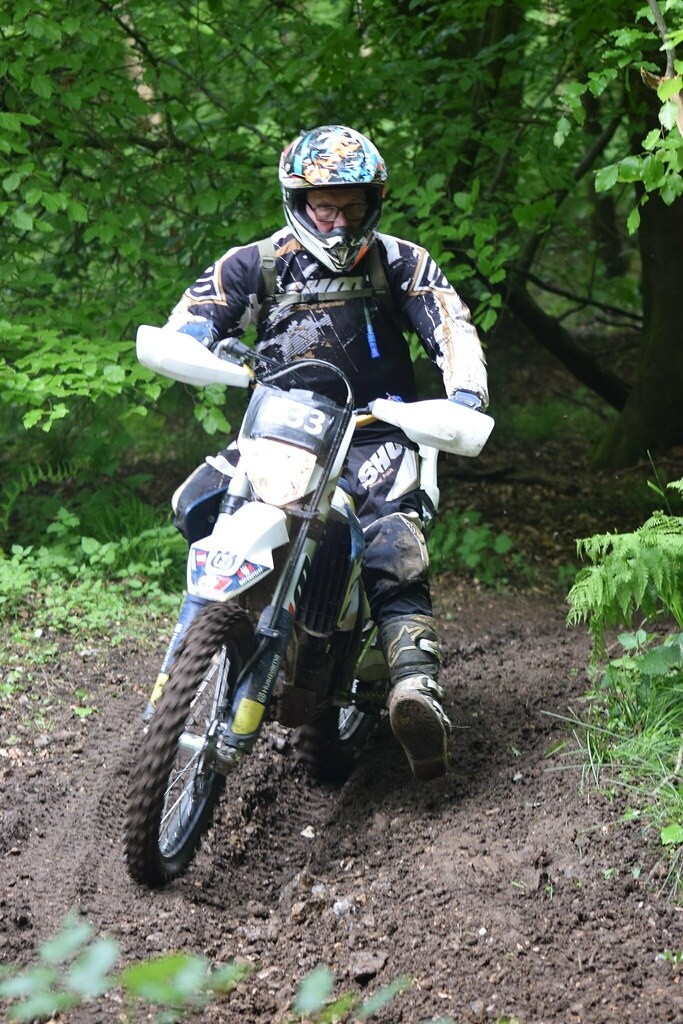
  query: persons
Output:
[158,122,491,783]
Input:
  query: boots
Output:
[375,613,450,782]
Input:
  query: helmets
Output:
[278,124,387,273]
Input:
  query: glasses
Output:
[304,199,370,222]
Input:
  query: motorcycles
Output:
[123,324,498,887]
